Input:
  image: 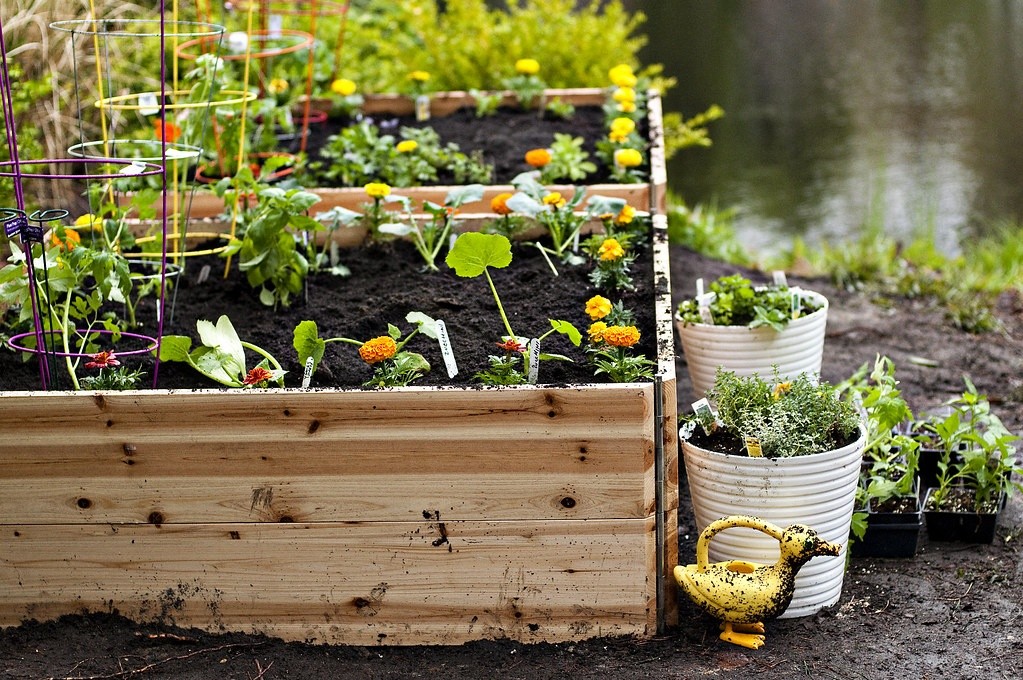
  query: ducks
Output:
[673,514,842,650]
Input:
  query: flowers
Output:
[677,361,865,457]
[0,57,657,386]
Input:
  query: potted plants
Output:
[675,273,830,413]
[834,352,1023,570]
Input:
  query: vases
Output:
[679,405,869,621]
[0,209,680,653]
[121,86,668,215]
